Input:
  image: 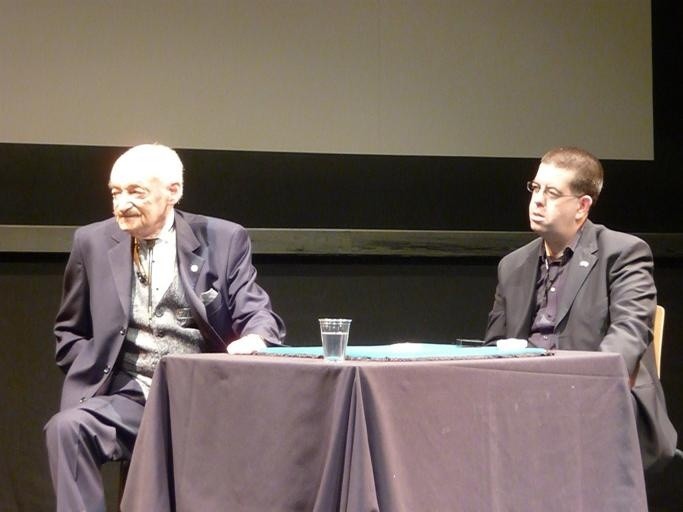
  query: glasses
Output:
[526,179,580,201]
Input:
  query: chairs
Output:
[652,305,667,380]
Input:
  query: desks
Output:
[120,342,649,512]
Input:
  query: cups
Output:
[318,318,354,363]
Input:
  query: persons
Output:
[39,139,289,512]
[486,142,678,477]
